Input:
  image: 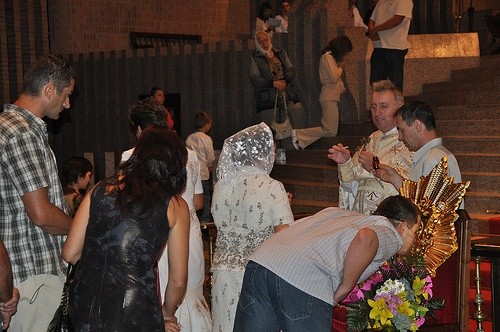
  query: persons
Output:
[275,0,292,36]
[248,31,308,153]
[48,125,190,332]
[254,3,276,38]
[62,84,220,332]
[230,194,423,332]
[365,0,412,94]
[291,34,353,151]
[1,54,79,332]
[326,80,420,215]
[1,241,20,332]
[373,101,465,216]
[211,124,297,332]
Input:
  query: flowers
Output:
[342,257,447,332]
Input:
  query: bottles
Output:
[373,156,380,170]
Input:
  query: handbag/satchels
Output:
[271,89,293,140]
[47,263,77,332]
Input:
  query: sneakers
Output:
[291,129,299,150]
